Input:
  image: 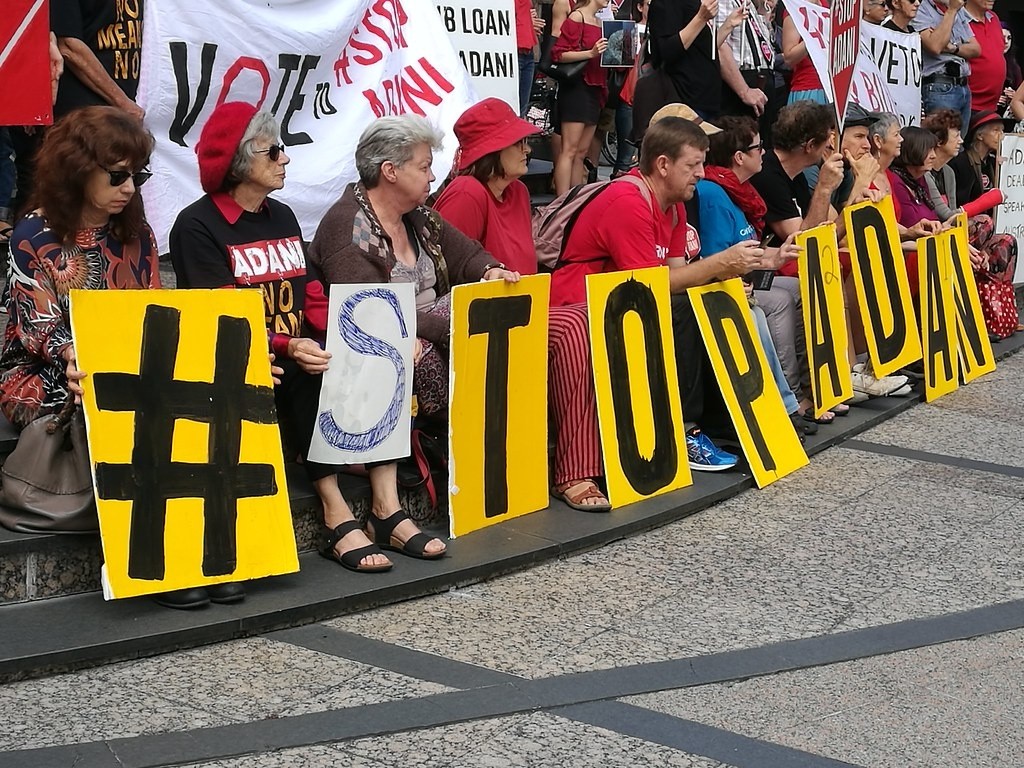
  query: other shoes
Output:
[789,359,925,445]
[989,332,1002,342]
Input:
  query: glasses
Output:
[97,163,153,188]
[910,0,923,4]
[251,144,285,161]
[871,2,890,7]
[731,140,763,157]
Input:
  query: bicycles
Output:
[601,129,639,168]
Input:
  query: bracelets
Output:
[950,42,959,55]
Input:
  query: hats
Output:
[195,101,259,193]
[649,103,726,136]
[450,98,545,179]
[844,101,881,126]
[963,110,1018,151]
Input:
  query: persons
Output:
[549,117,764,472]
[167,100,450,572]
[431,98,612,513]
[308,115,520,469]
[0,105,284,609]
[0,0,1024,445]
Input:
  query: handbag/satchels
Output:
[538,11,592,77]
[0,387,98,534]
[395,428,448,523]
[972,255,1019,338]
[619,40,654,108]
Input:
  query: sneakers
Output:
[685,425,739,471]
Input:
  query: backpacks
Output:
[532,175,676,270]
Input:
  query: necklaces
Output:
[879,171,891,192]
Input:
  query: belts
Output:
[923,75,967,87]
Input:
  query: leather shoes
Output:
[147,581,248,607]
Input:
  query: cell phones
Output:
[758,232,776,249]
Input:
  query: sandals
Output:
[549,476,612,513]
[0,228,14,243]
[317,520,393,573]
[362,509,447,559]
